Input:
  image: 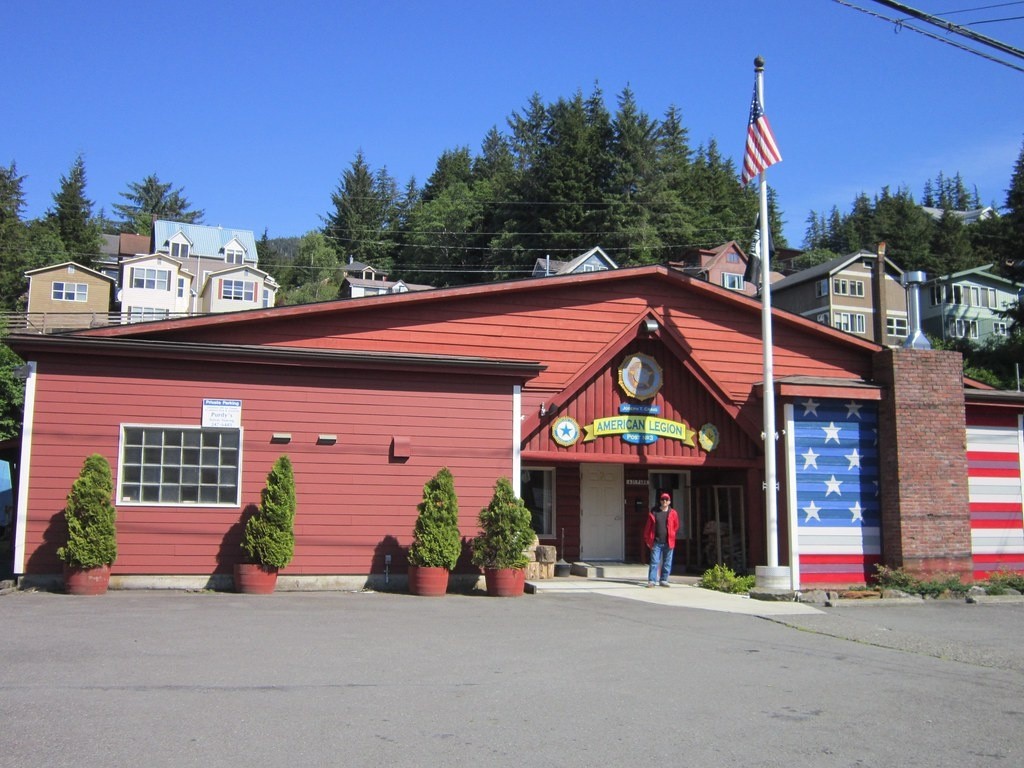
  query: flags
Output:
[744,209,776,296]
[741,80,783,185]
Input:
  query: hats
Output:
[661,493,670,501]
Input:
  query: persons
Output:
[643,493,680,588]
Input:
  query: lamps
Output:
[642,318,660,333]
[539,400,559,417]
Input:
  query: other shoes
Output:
[647,581,654,588]
[659,581,670,588]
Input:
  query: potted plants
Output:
[407,465,462,596]
[470,476,536,596]
[235,455,296,594]
[56,452,118,595]
